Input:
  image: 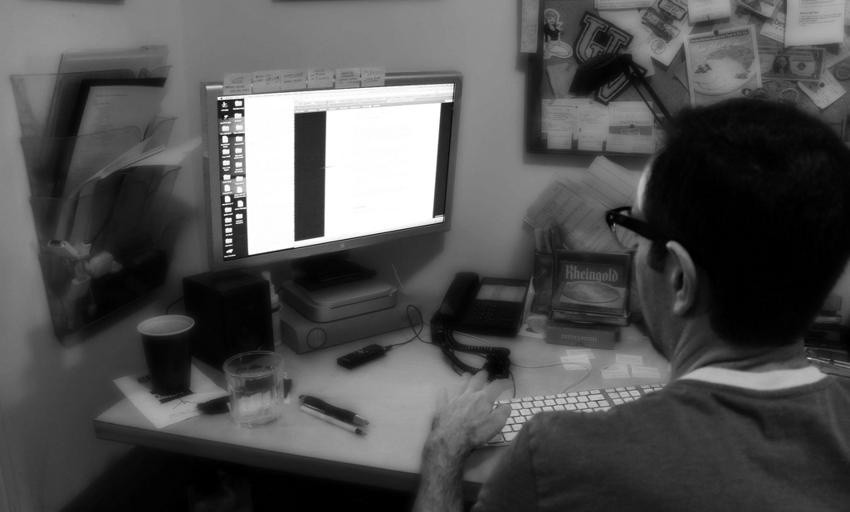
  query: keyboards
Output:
[482,383,669,446]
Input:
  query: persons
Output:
[413,95,849,512]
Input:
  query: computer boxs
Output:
[278,296,424,355]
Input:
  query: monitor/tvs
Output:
[200,70,463,322]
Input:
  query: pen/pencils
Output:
[299,395,370,433]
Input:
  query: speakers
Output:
[181,267,275,373]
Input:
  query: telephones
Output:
[431,272,528,336]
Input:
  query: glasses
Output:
[604,206,665,249]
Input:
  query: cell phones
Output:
[338,343,385,370]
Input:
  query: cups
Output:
[138,314,197,394]
[223,350,285,426]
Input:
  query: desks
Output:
[88,324,674,510]
